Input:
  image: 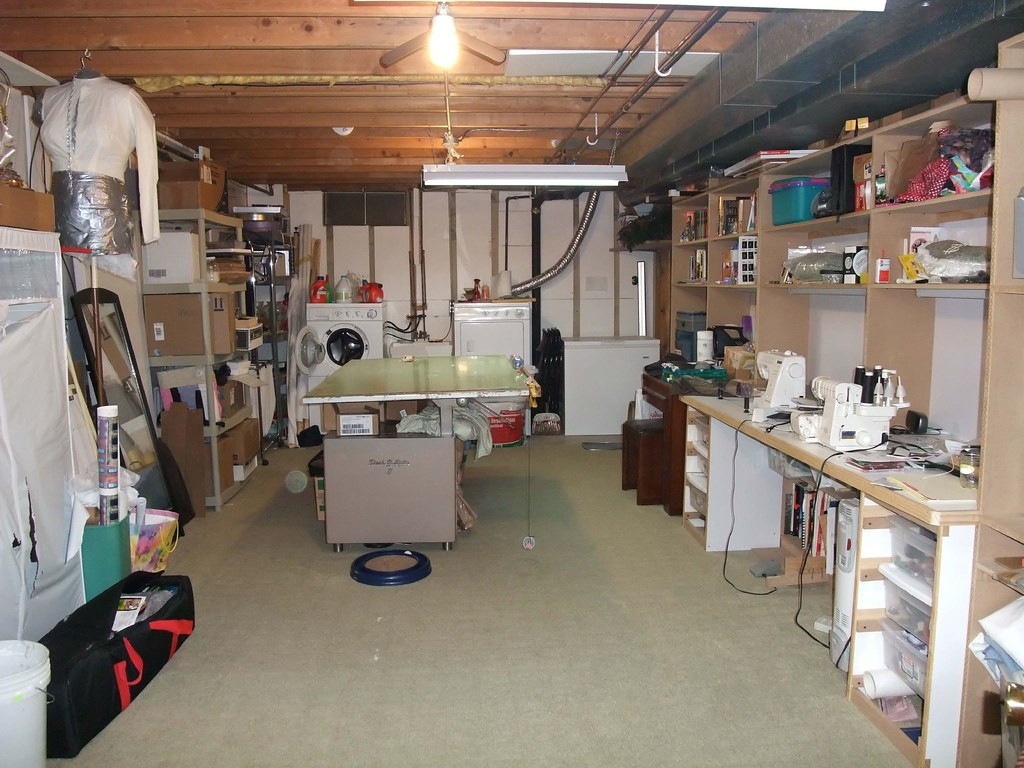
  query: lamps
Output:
[428,0,459,68]
[420,71,629,187]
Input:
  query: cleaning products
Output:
[335,269,385,303]
[309,274,332,303]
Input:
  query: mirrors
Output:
[69,286,186,543]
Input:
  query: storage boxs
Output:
[235,323,264,352]
[692,440,709,477]
[330,403,381,437]
[225,417,261,466]
[233,455,258,482]
[724,346,755,370]
[853,152,872,181]
[142,230,200,284]
[843,245,869,285]
[767,446,812,480]
[686,472,707,515]
[767,176,832,226]
[886,514,936,587]
[314,475,326,521]
[695,416,710,448]
[675,310,706,332]
[210,292,237,355]
[157,159,230,216]
[879,617,928,698]
[878,561,933,645]
[217,372,263,419]
[855,181,871,211]
[204,436,234,497]
[143,293,216,357]
[385,400,428,422]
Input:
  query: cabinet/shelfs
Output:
[669,92,995,430]
[954,32,1024,768]
[218,231,296,449]
[131,208,262,513]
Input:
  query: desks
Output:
[302,354,530,553]
[641,371,687,516]
[679,394,979,767]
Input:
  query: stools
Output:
[621,419,664,506]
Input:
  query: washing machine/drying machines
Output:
[292,301,390,435]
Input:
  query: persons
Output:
[30,68,160,258]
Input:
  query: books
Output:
[687,246,708,283]
[679,205,708,243]
[716,188,757,235]
[845,459,906,472]
[723,150,820,179]
[878,696,918,721]
[785,481,840,574]
[720,236,756,285]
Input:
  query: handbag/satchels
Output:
[127,507,180,574]
[34,570,194,757]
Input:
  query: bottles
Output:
[206,256,220,283]
[310,275,332,303]
[482,282,489,301]
[472,279,481,301]
[820,268,843,284]
[335,276,352,303]
[959,444,980,489]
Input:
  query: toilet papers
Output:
[490,270,512,298]
